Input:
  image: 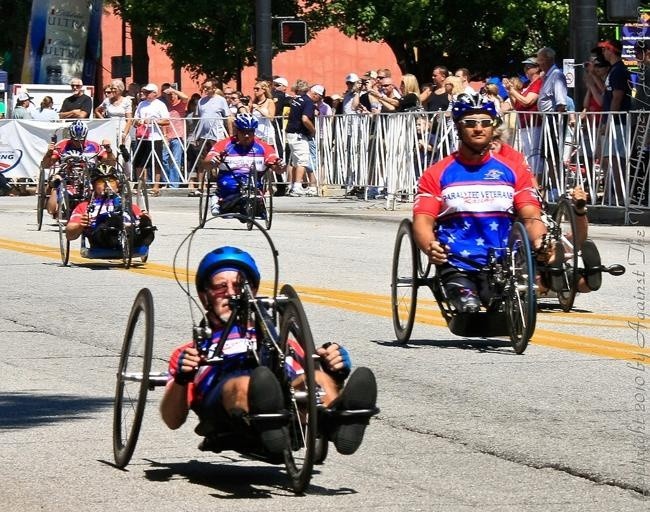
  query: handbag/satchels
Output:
[120,144,130,162]
[136,125,149,138]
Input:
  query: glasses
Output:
[104,91,111,94]
[377,76,386,79]
[459,118,493,126]
[381,84,391,87]
[71,85,82,88]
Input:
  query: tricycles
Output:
[105,217,380,495]
[54,159,158,269]
[521,153,627,313]
[386,189,567,355]
[197,150,276,230]
[34,128,134,234]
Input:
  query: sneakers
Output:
[141,214,154,245]
[345,185,387,197]
[285,184,318,197]
[540,242,564,291]
[581,239,602,291]
[248,366,287,453]
[328,368,377,455]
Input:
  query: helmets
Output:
[195,246,260,300]
[68,120,88,140]
[91,164,117,182]
[234,113,258,129]
[452,94,496,121]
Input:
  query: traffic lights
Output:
[276,18,307,46]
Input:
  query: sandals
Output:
[189,189,203,197]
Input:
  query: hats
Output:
[273,78,288,87]
[345,71,378,83]
[142,84,158,94]
[597,40,622,52]
[309,84,326,100]
[17,93,34,103]
[521,57,538,65]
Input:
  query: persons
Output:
[65,163,154,248]
[530,176,602,299]
[0,39,650,215]
[159,245,377,465]
[411,93,556,314]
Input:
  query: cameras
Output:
[361,81,368,85]
[240,96,251,104]
[583,62,593,68]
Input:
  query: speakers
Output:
[111,56,131,79]
